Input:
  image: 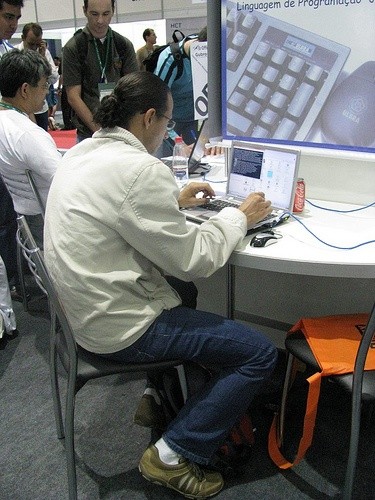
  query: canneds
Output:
[293,178,305,212]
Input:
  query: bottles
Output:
[171,137,189,186]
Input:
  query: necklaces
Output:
[0,103,27,115]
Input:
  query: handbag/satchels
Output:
[267,313,375,470]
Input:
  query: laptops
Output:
[178,140,301,236]
[161,118,208,177]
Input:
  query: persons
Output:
[44,72,271,500]
[0,50,62,250]
[0,0,23,60]
[137,29,157,70]
[14,23,60,131]
[153,26,225,155]
[62,0,137,143]
[47,84,57,131]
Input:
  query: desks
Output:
[162,153,375,332]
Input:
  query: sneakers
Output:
[137,445,225,500]
[128,394,161,429]
[48,116,57,131]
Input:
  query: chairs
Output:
[277,302,375,500]
[0,169,47,313]
[15,216,189,500]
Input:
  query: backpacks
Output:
[142,29,192,82]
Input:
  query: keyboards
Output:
[226,1,351,136]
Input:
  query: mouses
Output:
[250,231,282,248]
[321,60,375,148]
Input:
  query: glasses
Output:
[140,107,177,131]
